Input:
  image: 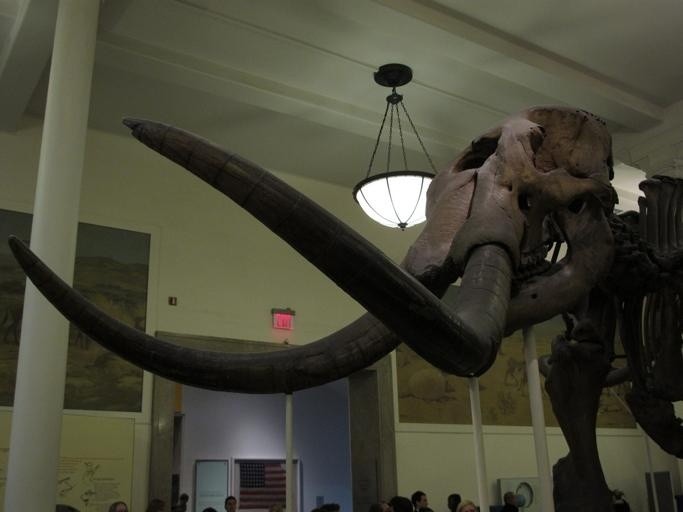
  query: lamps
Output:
[349,62,440,233]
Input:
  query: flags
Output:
[238,463,286,509]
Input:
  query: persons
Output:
[447,491,519,511]
[612,488,631,512]
[109,493,237,511]
[312,491,433,511]
